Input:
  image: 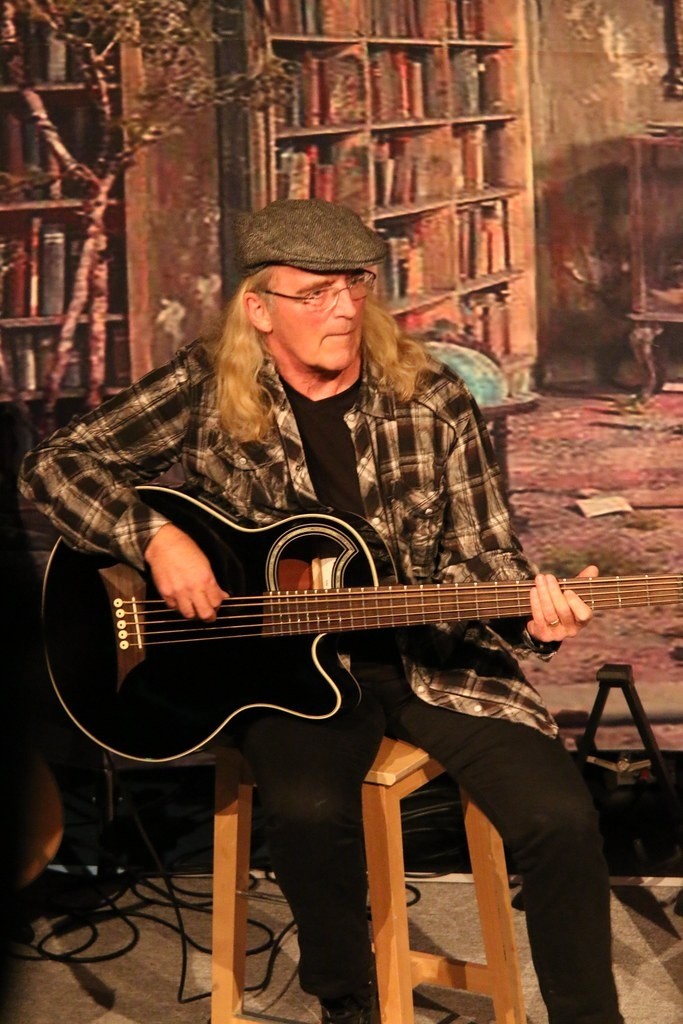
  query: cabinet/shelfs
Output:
[225,0,539,396]
[0,1,152,405]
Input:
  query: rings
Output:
[550,619,559,627]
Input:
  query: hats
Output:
[233,197,387,273]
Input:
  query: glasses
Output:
[257,268,377,315]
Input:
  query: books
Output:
[0,0,131,397]
[264,0,512,365]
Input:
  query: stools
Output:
[205,736,527,1023]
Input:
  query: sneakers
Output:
[321,951,376,1024]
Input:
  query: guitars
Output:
[40,484,683,766]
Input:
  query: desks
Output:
[624,128,682,407]
[482,392,543,511]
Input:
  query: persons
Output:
[17,198,626,1024]
